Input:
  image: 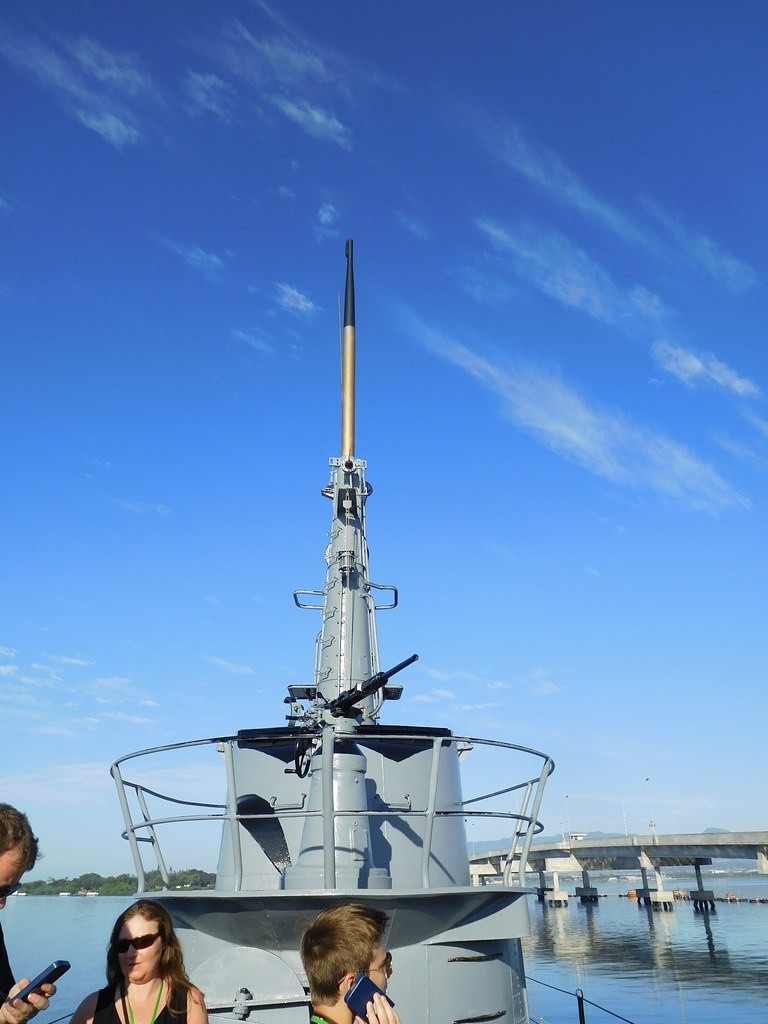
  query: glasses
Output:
[338,951,392,984]
[0,883,21,901]
[115,931,160,953]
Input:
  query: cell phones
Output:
[9,961,71,1008]
[346,973,395,1024]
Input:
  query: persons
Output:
[299,904,400,1024]
[69,899,210,1024]
[0,803,58,1024]
